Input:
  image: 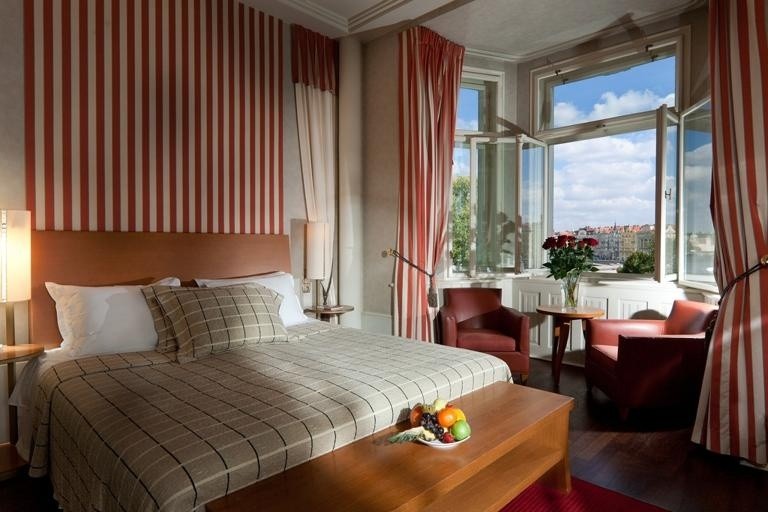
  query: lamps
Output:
[1,207,31,446]
[304,221,332,317]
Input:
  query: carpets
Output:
[499,470,673,511]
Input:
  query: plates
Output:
[419,436,470,450]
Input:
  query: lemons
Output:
[453,408,466,421]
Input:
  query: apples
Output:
[451,419,471,441]
[410,405,423,426]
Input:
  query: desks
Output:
[204,383,576,512]
[306,302,355,323]
[0,342,44,483]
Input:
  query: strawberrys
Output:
[442,432,454,443]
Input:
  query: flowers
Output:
[539,232,601,294]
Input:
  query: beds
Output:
[29,317,513,512]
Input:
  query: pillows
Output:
[45,270,315,364]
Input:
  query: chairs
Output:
[436,287,530,382]
[582,299,722,421]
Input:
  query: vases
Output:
[560,274,579,309]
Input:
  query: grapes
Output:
[418,413,444,442]
